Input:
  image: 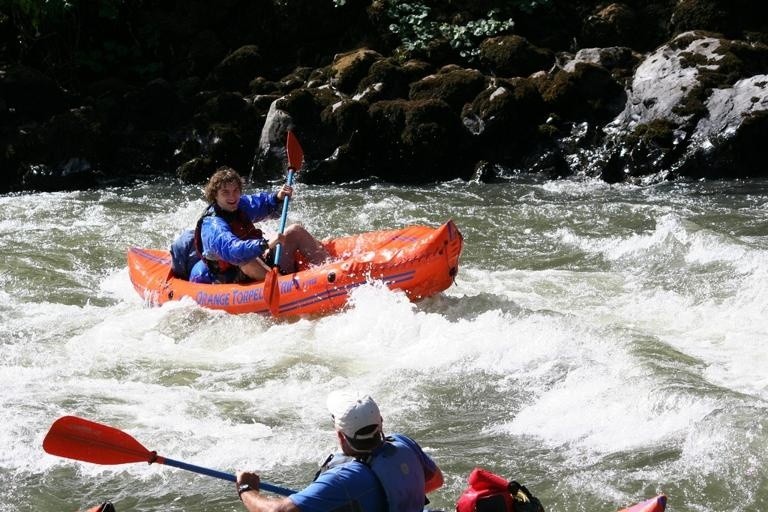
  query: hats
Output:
[331,394,384,440]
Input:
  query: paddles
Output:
[42,414,299,495]
[264,131,304,318]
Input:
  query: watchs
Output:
[239,484,252,500]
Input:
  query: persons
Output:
[234,393,444,512]
[187,167,330,282]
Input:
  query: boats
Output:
[124,216,468,322]
[86,492,669,512]
